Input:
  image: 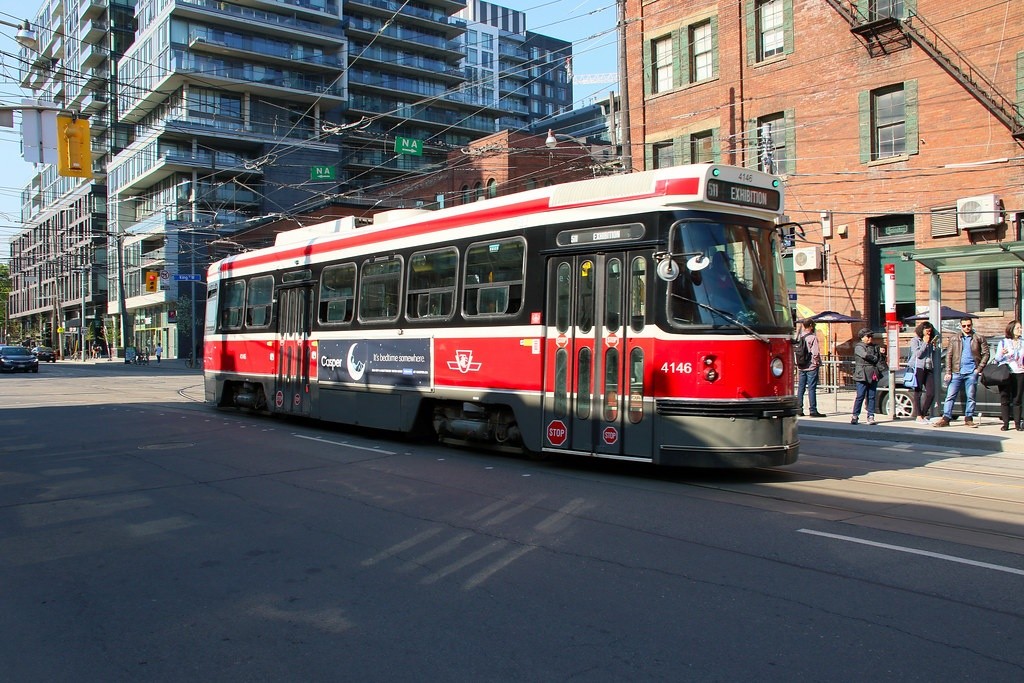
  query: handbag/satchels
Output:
[980,339,1011,386]
[902,371,918,387]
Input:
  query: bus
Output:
[200,164,810,470]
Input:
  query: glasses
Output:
[864,335,873,338]
[961,324,972,328]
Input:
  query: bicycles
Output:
[129,346,149,365]
[185,352,202,369]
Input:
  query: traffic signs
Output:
[173,274,201,281]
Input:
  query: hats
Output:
[858,327,874,338]
[712,250,734,264]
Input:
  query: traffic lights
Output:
[146,272,158,293]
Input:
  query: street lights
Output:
[546,89,618,176]
[80,262,93,361]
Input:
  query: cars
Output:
[0,346,39,373]
[867,333,1024,423]
[31,347,57,363]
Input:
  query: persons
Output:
[851,328,884,425]
[906,321,939,426]
[795,318,826,417]
[154,343,163,366]
[91,340,102,360]
[703,251,755,312]
[931,316,991,428]
[994,319,1024,432]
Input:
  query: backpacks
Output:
[795,333,815,368]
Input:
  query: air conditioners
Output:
[792,246,822,272]
[956,194,1001,229]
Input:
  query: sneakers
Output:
[867,415,876,425]
[915,416,933,424]
[932,417,950,427]
[850,415,859,424]
[965,417,978,428]
